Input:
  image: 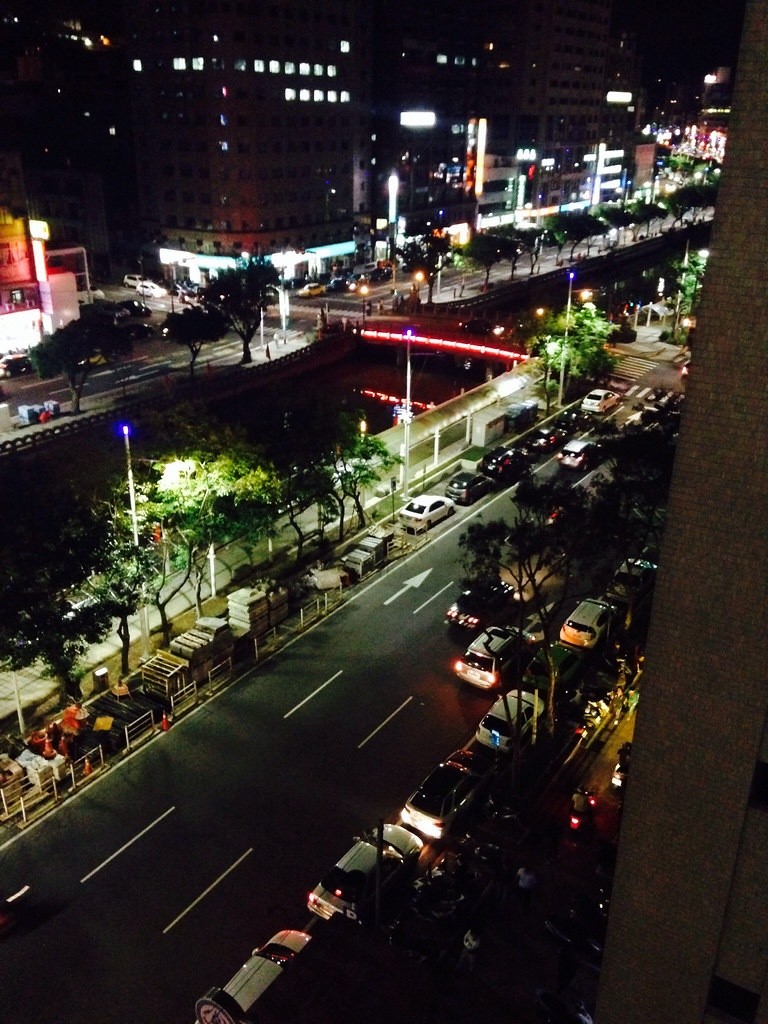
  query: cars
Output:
[224,929,318,1021]
[442,578,515,629]
[527,426,568,452]
[458,317,505,337]
[297,258,391,298]
[523,557,658,691]
[305,825,426,930]
[555,387,623,473]
[444,470,495,506]
[0,274,232,381]
[400,747,489,841]
[475,689,546,753]
[398,494,454,532]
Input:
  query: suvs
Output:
[453,624,536,691]
[480,447,534,486]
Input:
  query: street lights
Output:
[360,286,368,331]
[415,272,423,312]
[558,270,577,405]
[401,325,416,486]
[121,424,141,575]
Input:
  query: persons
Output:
[458,927,482,969]
[572,785,588,812]
[516,865,536,913]
[612,684,640,725]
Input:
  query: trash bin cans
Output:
[92,666,110,692]
